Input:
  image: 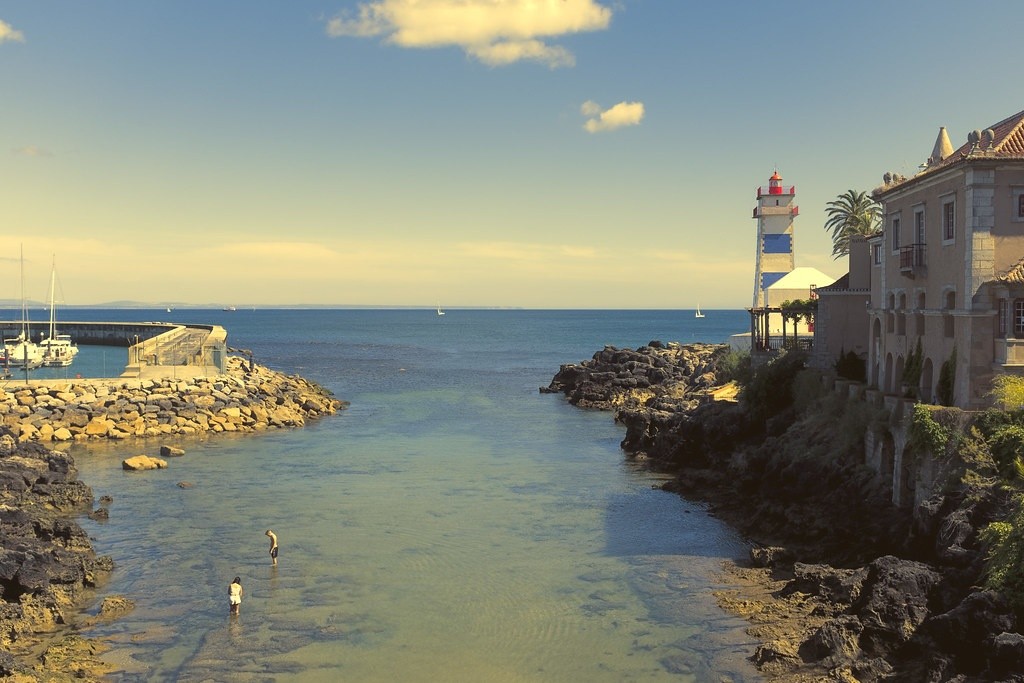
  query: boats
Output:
[222,308,228,311]
[229,307,236,311]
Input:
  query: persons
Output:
[228,577,242,614]
[265,530,279,564]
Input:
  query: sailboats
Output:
[167,307,171,312]
[438,304,445,315]
[0,242,80,367]
[695,304,705,318]
